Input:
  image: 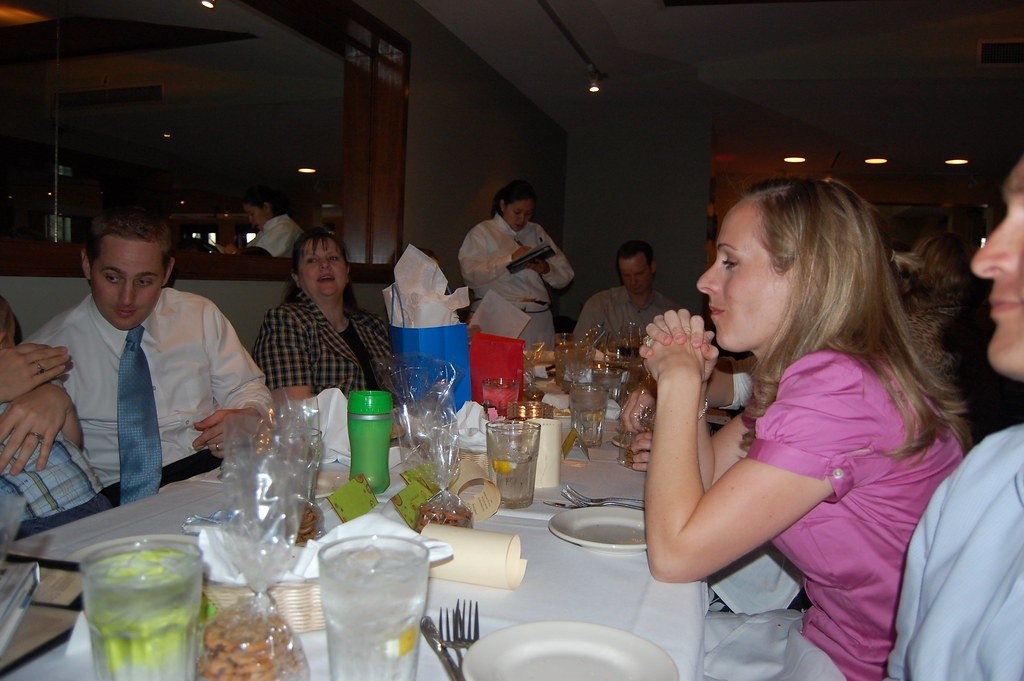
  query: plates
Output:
[462,620,682,681]
[554,415,572,419]
[548,505,648,555]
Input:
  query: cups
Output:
[555,333,573,345]
[617,430,647,471]
[570,384,608,449]
[481,379,520,424]
[554,343,595,394]
[485,420,540,509]
[592,368,623,408]
[78,535,203,681]
[318,535,430,681]
[620,322,641,358]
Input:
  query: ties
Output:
[117,325,163,505]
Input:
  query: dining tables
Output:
[0,321,848,681]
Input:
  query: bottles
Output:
[282,427,322,502]
[347,390,393,494]
[46,166,83,245]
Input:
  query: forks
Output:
[455,598,480,649]
[439,607,464,672]
[561,485,645,511]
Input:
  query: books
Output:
[505,244,555,274]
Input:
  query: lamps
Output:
[588,75,600,93]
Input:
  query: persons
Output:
[573,239,678,352]
[383,247,483,345]
[881,160,1024,681]
[617,177,972,681]
[0,296,114,541]
[18,204,271,508]
[459,178,574,366]
[250,227,406,440]
[224,176,304,258]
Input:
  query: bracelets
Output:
[697,397,708,420]
[231,248,238,255]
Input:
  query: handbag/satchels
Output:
[388,283,472,415]
[469,331,526,417]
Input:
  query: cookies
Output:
[295,505,316,540]
[416,502,474,531]
[195,607,304,681]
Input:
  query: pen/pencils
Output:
[513,234,524,250]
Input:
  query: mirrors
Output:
[0,0,412,284]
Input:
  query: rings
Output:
[27,432,44,440]
[216,444,221,451]
[642,335,654,348]
[621,408,625,412]
[33,360,44,373]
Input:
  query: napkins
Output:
[200,474,454,584]
[454,400,491,451]
[272,387,411,469]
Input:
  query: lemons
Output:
[386,626,416,657]
[492,458,518,475]
